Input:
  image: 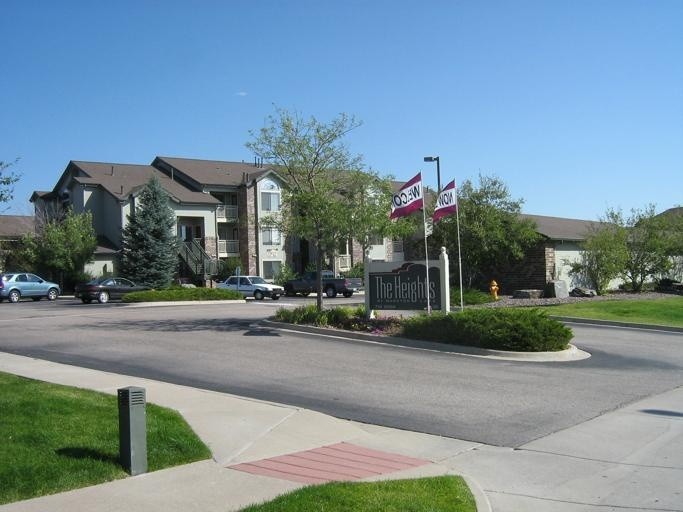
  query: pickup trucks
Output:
[283,270,362,298]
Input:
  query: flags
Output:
[388,173,422,219]
[431,179,457,220]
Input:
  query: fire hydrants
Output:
[488,280,499,302]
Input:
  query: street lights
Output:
[423,156,442,260]
[356,184,372,287]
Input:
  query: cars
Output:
[0,272,60,303]
[74,276,155,304]
[215,275,284,300]
